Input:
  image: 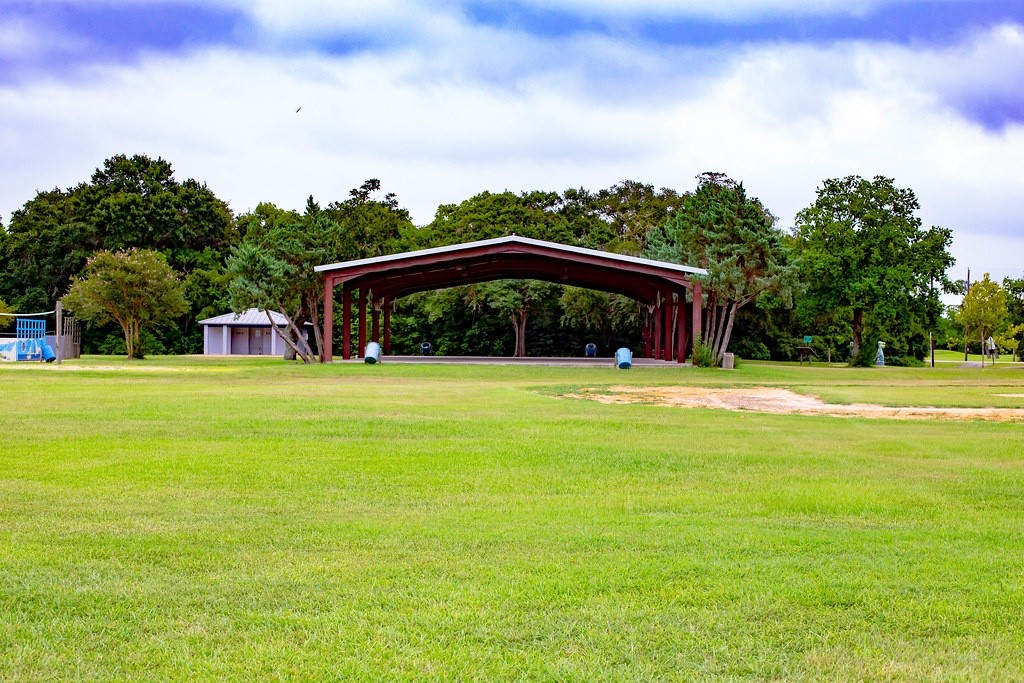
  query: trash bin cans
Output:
[42,344,56,362]
[364,342,380,364]
[421,342,431,356]
[586,343,596,357]
[722,353,735,369]
[616,348,631,369]
